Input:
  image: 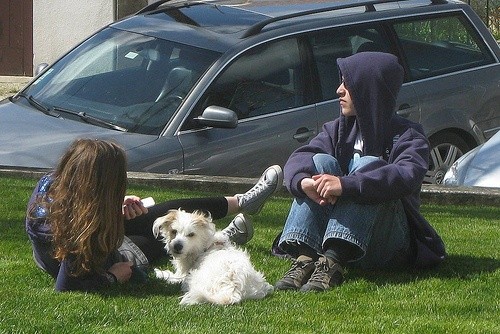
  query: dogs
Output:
[152,207,275,308]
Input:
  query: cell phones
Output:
[122,197,155,216]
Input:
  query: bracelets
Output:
[105,271,118,285]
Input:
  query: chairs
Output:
[154,66,194,101]
[228,81,297,117]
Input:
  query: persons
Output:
[25,138,284,291]
[275,52,445,292]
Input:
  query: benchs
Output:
[319,30,483,101]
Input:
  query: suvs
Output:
[0,0,500,179]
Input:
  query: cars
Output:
[440,129,500,188]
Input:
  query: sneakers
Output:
[222,213,254,245]
[275,255,316,291]
[234,165,284,215]
[298,252,347,293]
